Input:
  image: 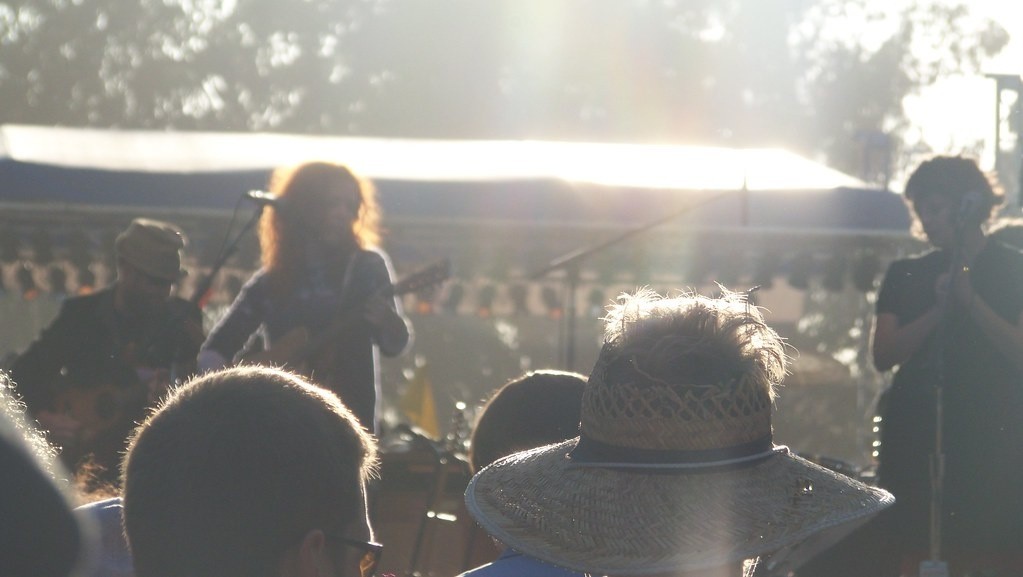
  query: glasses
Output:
[325,533,383,577]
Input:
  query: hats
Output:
[464,335,895,574]
[116,217,188,279]
[75,497,134,574]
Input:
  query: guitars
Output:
[238,257,453,384]
[34,371,166,459]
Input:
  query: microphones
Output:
[243,189,283,208]
[739,184,749,226]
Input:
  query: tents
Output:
[1,124,915,464]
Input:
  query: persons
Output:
[0,369,373,577]
[9,223,206,502]
[872,156,1023,577]
[449,300,894,577]
[198,161,407,503]
